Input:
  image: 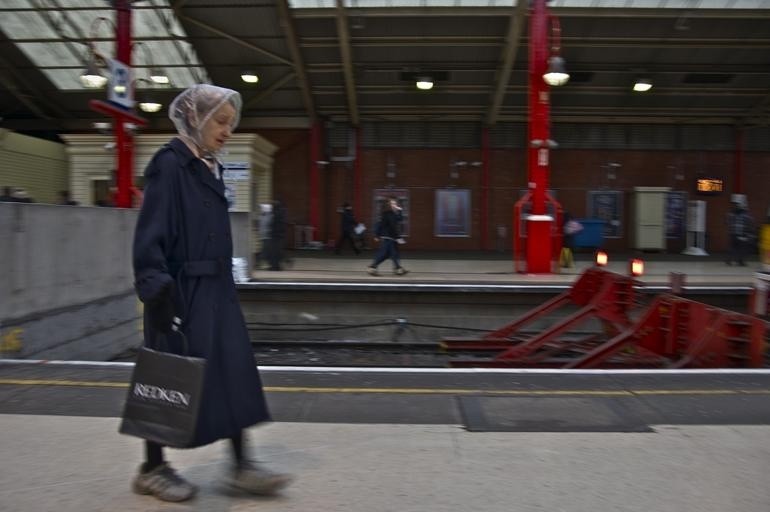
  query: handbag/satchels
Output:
[117,317,208,448]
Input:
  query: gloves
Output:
[147,290,177,333]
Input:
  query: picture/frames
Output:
[518,189,559,237]
[433,187,472,239]
[664,190,689,240]
[370,188,412,239]
[586,189,624,240]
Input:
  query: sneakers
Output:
[131,461,197,502]
[218,461,292,494]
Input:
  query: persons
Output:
[724,194,758,266]
[130,84,295,502]
[335,193,409,276]
[0,185,118,208]
[254,198,295,272]
